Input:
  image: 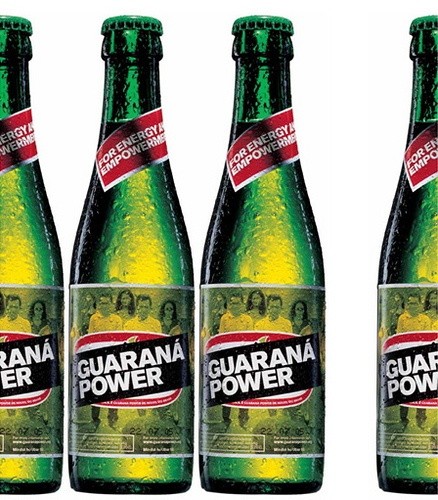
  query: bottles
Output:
[0,14,63,494]
[194,14,326,495]
[67,13,194,494]
[376,16,438,494]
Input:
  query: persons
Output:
[386,294,405,335]
[78,294,97,337]
[0,292,32,335]
[219,293,253,430]
[210,291,228,334]
[255,295,291,416]
[423,290,437,327]
[288,301,314,425]
[396,291,432,433]
[245,289,268,321]
[28,299,49,337]
[113,289,135,320]
[157,301,180,423]
[86,293,123,434]
[124,295,161,419]
